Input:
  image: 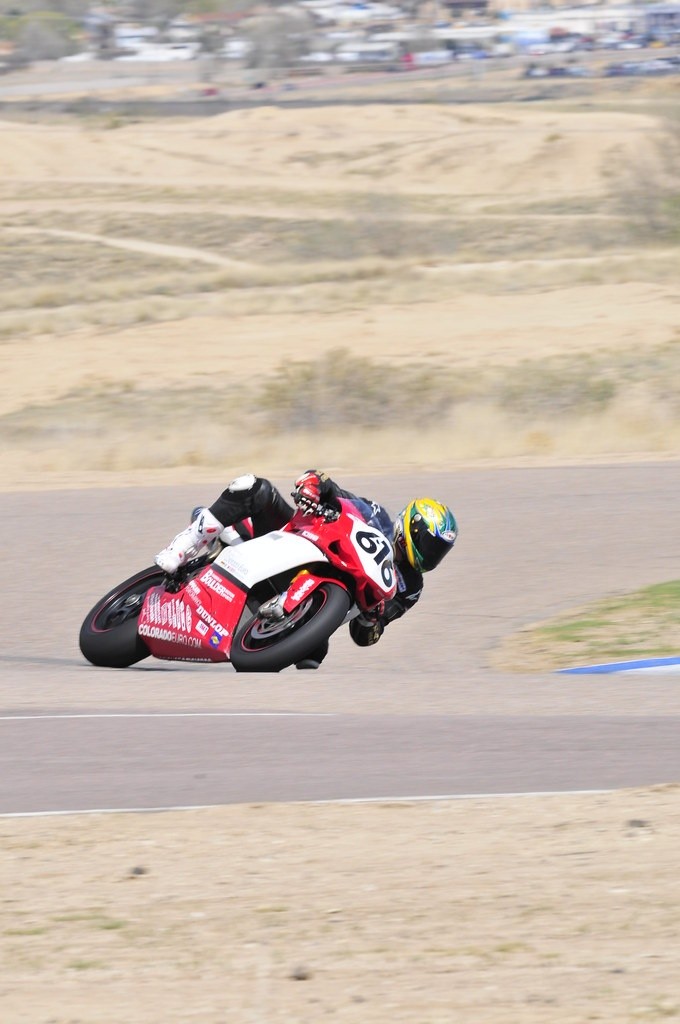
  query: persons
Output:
[152,470,458,670]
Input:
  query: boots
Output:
[153,508,225,573]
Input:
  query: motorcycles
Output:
[77,494,397,675]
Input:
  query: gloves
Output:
[295,475,321,518]
[364,599,386,622]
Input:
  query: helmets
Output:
[393,498,457,573]
